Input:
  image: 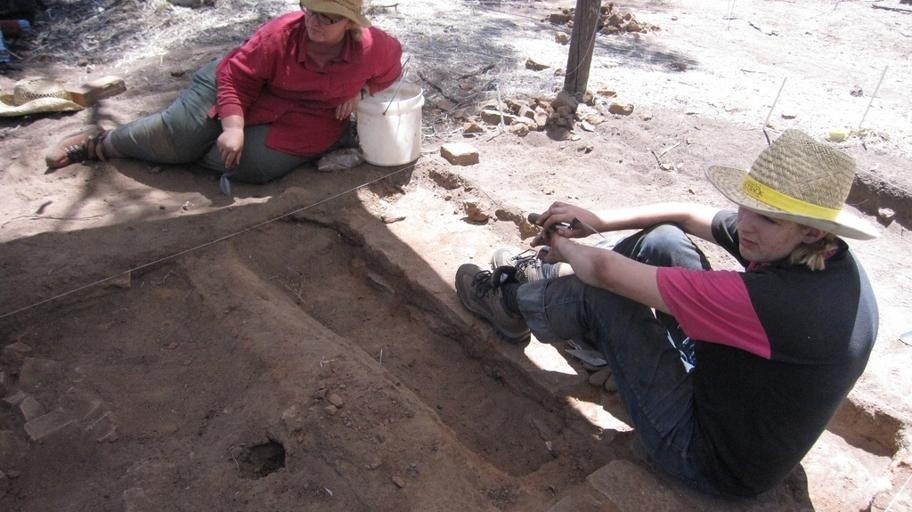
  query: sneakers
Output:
[45,125,108,171]
[492,246,546,285]
[455,261,538,346]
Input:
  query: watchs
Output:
[359,86,368,98]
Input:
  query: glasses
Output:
[300,3,344,25]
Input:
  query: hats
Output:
[0,76,85,118]
[699,125,881,244]
[299,0,372,28]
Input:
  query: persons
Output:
[454,129,879,497]
[44,0,401,186]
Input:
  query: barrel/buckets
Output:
[356,58,423,166]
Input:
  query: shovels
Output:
[219,166,239,202]
[528,213,608,241]
